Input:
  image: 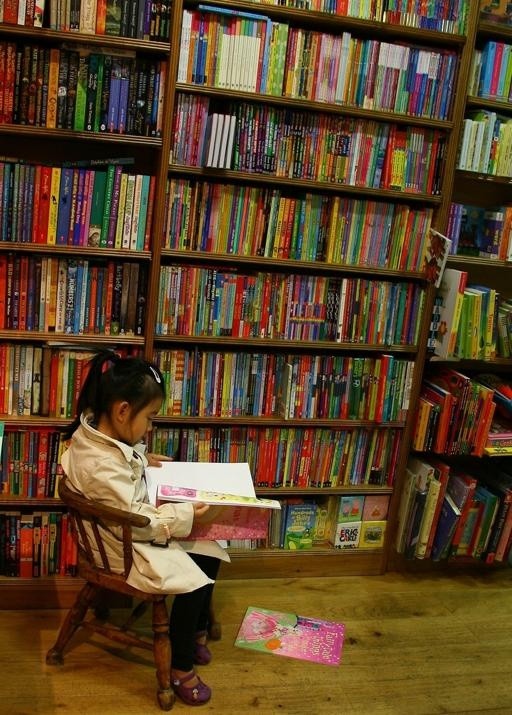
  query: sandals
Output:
[188,640,211,664]
[156,665,211,705]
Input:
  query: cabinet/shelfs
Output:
[2,2,182,609]
[387,2,512,576]
[145,1,478,581]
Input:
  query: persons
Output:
[59,350,234,704]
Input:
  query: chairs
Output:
[46,475,183,710]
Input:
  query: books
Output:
[234,606,347,665]
